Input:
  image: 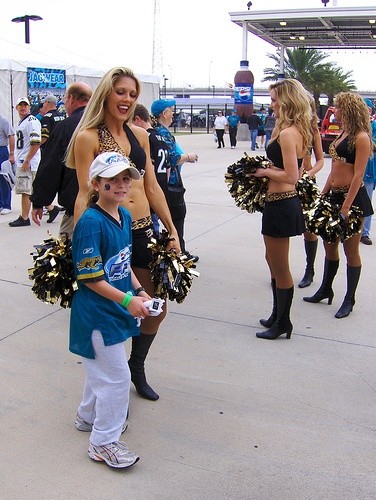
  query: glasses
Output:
[39,103,44,108]
[165,109,175,113]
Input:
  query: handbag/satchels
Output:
[214,134,217,143]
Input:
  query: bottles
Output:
[233,61,254,123]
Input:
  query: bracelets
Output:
[120,293,133,308]
[134,287,146,296]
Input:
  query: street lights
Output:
[11,14,43,44]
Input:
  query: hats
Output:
[89,153,140,180]
[16,97,30,106]
[151,100,176,115]
[231,109,237,112]
[364,99,372,108]
[41,95,57,105]
[218,110,222,112]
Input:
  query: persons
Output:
[0,116,16,215]
[246,106,276,151]
[8,95,67,228]
[148,99,200,263]
[30,81,94,254]
[303,92,373,320]
[247,78,312,340]
[227,109,241,149]
[296,93,324,289]
[360,98,376,246]
[72,65,182,402]
[68,150,153,471]
[214,110,227,148]
[132,104,170,263]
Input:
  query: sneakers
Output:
[87,440,140,468]
[9,215,30,227]
[47,206,59,223]
[75,414,128,434]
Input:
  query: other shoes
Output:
[57,207,66,214]
[44,207,49,215]
[361,235,372,245]
[0,208,11,215]
[184,252,199,262]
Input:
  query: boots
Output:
[127,333,160,401]
[260,278,278,329]
[256,284,294,339]
[335,262,361,318]
[298,238,318,288]
[303,256,339,305]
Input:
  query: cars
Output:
[320,105,343,154]
